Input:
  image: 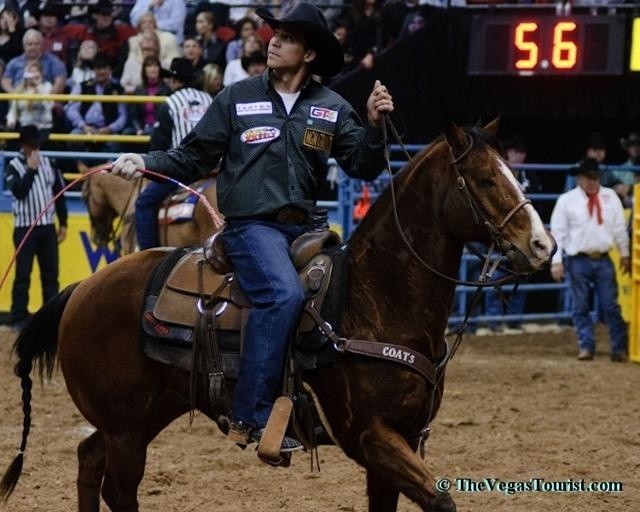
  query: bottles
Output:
[89,0,115,15]
[9,125,51,149]
[162,56,202,85]
[563,157,609,179]
[253,3,344,78]
[87,52,117,70]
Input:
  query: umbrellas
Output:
[228,414,303,455]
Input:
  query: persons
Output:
[562,143,630,315]
[613,129,640,195]
[105,2,397,458]
[476,143,533,334]
[0,2,431,172]
[132,57,220,255]
[3,124,71,329]
[545,157,631,363]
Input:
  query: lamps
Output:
[44,22,275,62]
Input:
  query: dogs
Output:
[0,116,557,512]
[75,158,227,257]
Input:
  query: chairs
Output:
[610,350,630,364]
[576,348,595,363]
